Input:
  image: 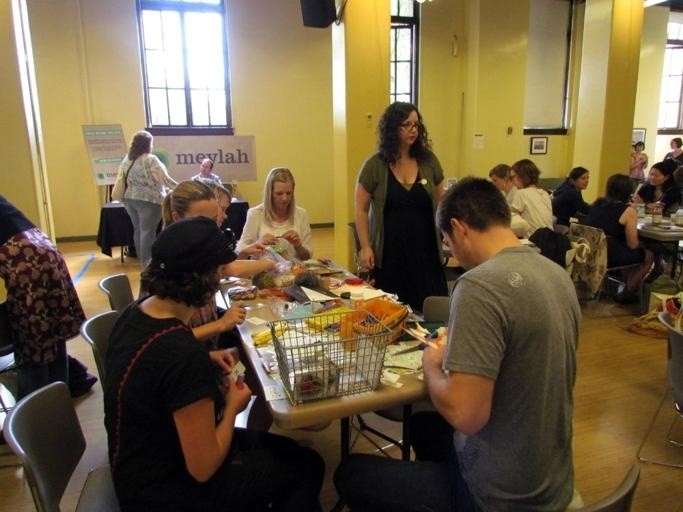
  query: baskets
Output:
[269,309,393,405]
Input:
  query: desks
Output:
[637,214,683,280]
[96,196,249,263]
[219,258,446,511]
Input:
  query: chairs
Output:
[529,223,660,319]
[0,273,135,512]
[346,222,375,281]
[564,464,641,512]
[636,311,683,468]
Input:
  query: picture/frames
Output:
[632,128,646,148]
[530,136,548,155]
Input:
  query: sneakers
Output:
[615,290,636,304]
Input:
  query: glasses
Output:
[401,121,420,129]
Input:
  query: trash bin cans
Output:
[657,310,683,413]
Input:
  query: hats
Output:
[151,215,238,270]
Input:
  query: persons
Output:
[102,215,327,512]
[234,166,313,260]
[352,100,449,315]
[189,158,223,193]
[156,180,246,351]
[205,181,276,281]
[331,174,582,511]
[482,138,682,305]
[111,130,180,270]
[0,193,87,408]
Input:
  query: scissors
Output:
[393,331,441,356]
